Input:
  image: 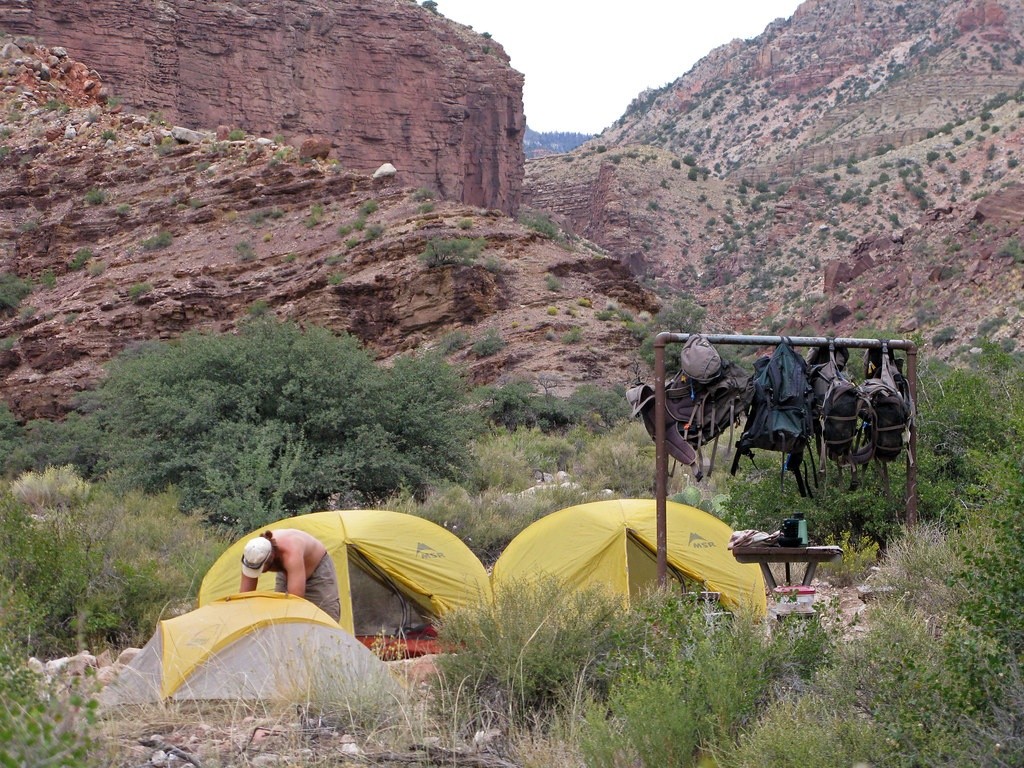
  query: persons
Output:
[239,527,340,624]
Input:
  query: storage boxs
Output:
[773,586,817,612]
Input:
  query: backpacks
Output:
[730,336,819,497]
[627,358,753,481]
[803,338,878,491]
[856,340,915,470]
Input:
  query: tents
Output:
[92,498,767,709]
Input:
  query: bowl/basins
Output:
[777,536,804,548]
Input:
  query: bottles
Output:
[789,512,809,547]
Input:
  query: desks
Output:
[733,546,843,621]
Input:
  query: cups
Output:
[781,519,799,538]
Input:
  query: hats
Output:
[682,334,721,383]
[241,537,272,578]
